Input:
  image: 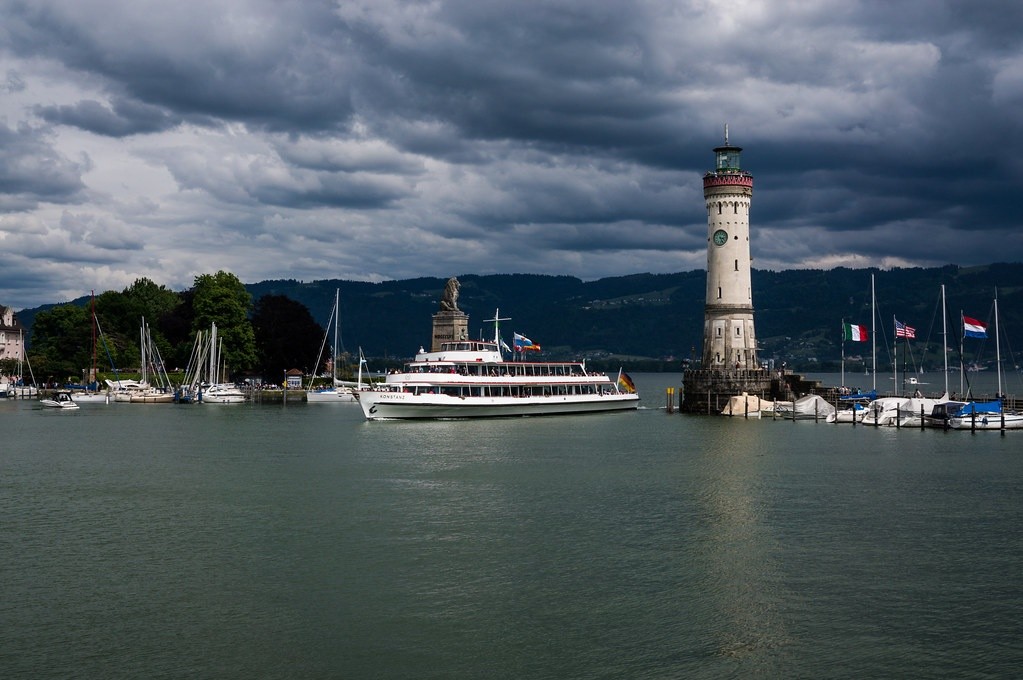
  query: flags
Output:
[514,340,540,352]
[843,323,868,341]
[514,333,532,346]
[962,316,989,338]
[896,321,915,337]
[500,340,511,352]
[619,370,635,392]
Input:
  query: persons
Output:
[362,345,614,400]
[915,390,922,397]
[780,362,785,370]
[736,361,740,370]
[833,385,861,395]
[951,392,957,401]
[942,391,946,397]
[255,382,332,391]
[996,390,1006,398]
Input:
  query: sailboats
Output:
[0,286,391,404]
[824,270,1023,429]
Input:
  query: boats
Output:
[39,392,80,410]
[350,308,641,421]
